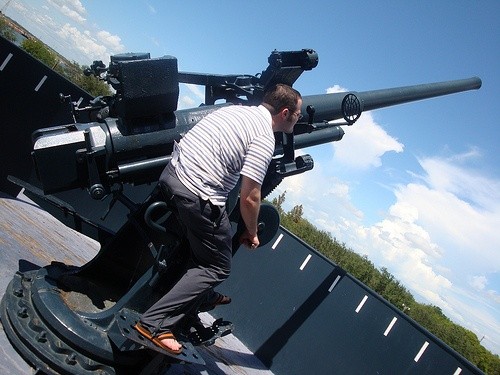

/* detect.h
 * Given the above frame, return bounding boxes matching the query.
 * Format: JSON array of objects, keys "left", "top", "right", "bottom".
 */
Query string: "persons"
[{"left": 134, "top": 84, "right": 303, "bottom": 354}]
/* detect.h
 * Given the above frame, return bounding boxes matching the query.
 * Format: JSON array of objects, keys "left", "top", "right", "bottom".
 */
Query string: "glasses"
[{"left": 288, "top": 109, "right": 303, "bottom": 120}]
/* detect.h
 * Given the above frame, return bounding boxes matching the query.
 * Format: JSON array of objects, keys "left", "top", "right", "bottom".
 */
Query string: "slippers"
[{"left": 134, "top": 321, "right": 183, "bottom": 354}]
